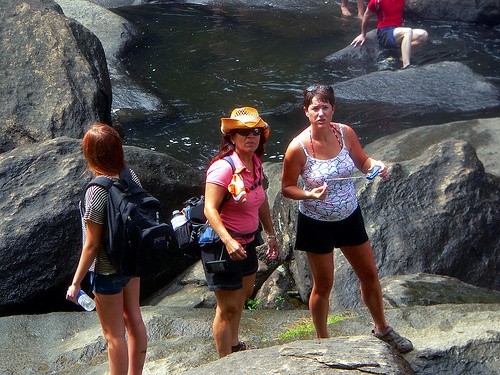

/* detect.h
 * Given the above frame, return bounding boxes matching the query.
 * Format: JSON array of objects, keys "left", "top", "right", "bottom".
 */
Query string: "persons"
[
  {"left": 341, "top": 1, "right": 364, "bottom": 20},
  {"left": 282, "top": 87, "right": 412, "bottom": 354},
  {"left": 66, "top": 124, "right": 148, "bottom": 375},
  {"left": 199, "top": 107, "right": 278, "bottom": 360},
  {"left": 351, "top": 0, "right": 428, "bottom": 70}
]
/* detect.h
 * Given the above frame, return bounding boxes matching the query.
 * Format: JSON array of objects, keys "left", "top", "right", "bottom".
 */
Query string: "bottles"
[
  {"left": 172, "top": 210, "right": 182, "bottom": 219},
  {"left": 68, "top": 285, "right": 96, "bottom": 312}
]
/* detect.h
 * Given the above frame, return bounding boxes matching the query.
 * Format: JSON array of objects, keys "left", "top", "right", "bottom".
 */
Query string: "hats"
[{"left": 221, "top": 107, "right": 270, "bottom": 140}]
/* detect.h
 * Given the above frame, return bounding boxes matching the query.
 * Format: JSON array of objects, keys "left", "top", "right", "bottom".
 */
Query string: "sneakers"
[{"left": 372, "top": 326, "right": 413, "bottom": 354}]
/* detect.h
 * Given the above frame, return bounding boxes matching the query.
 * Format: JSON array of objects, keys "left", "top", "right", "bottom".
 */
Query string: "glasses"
[{"left": 236, "top": 128, "right": 261, "bottom": 136}]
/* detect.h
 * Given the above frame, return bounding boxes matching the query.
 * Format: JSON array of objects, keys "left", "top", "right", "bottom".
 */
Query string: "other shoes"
[
  {"left": 232, "top": 342, "right": 257, "bottom": 352},
  {"left": 401, "top": 64, "right": 419, "bottom": 70}
]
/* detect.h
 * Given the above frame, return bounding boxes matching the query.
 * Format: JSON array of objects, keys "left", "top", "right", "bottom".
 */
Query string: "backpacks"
[
  {"left": 169, "top": 155, "right": 264, "bottom": 248},
  {"left": 82, "top": 166, "right": 170, "bottom": 274}
]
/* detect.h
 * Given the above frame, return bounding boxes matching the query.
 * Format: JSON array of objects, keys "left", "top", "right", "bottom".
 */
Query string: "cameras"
[{"left": 366, "top": 166, "right": 381, "bottom": 180}]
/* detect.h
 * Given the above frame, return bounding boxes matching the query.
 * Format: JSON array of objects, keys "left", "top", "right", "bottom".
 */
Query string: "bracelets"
[{"left": 266, "top": 235, "right": 276, "bottom": 238}]
